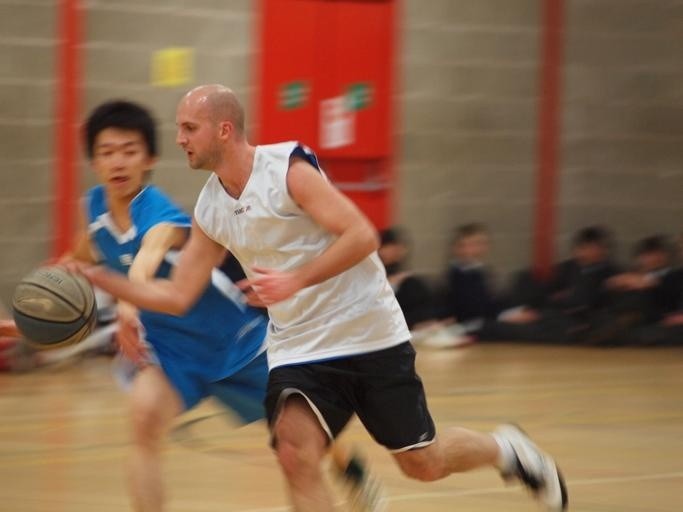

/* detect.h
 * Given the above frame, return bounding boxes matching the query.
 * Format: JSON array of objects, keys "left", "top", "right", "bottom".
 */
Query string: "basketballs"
[{"left": 13, "top": 265, "right": 97, "bottom": 350}]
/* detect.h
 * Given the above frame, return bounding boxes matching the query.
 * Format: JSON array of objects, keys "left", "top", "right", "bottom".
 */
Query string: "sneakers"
[{"left": 497, "top": 422, "right": 566, "bottom": 512}]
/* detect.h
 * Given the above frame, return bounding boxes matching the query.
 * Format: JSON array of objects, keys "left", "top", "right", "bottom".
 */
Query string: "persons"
[
  {"left": 46, "top": 84, "right": 567, "bottom": 512},
  {"left": 378, "top": 224, "right": 683, "bottom": 348},
  {"left": 0, "top": 101, "right": 381, "bottom": 512}
]
